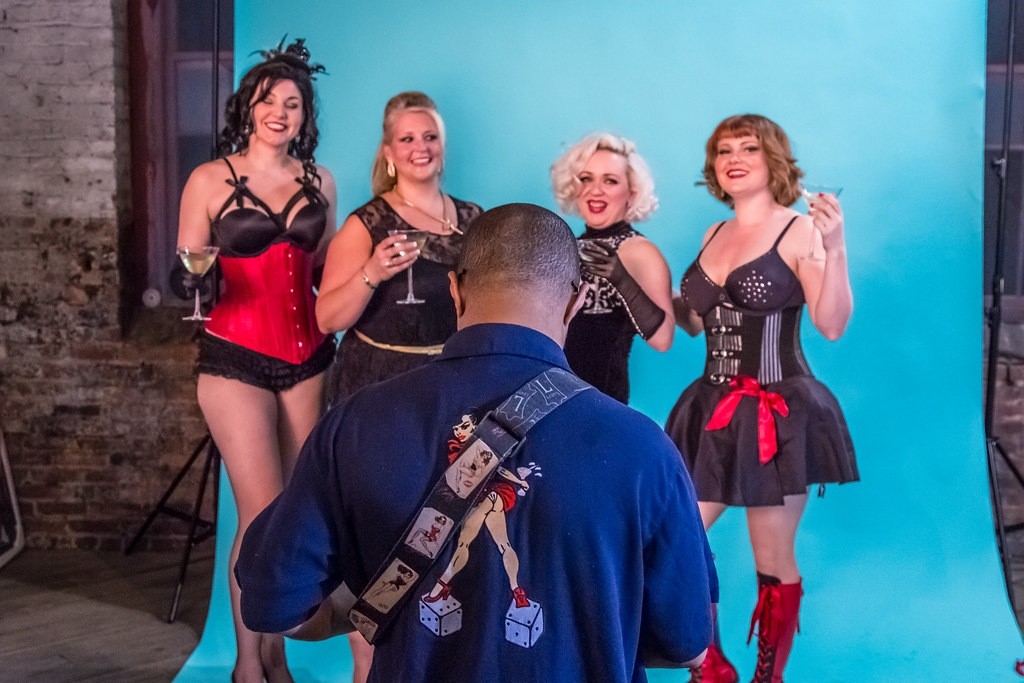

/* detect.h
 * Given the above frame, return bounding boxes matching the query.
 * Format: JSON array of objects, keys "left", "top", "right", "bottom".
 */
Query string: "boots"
[
  {"left": 747, "top": 571, "right": 803, "bottom": 683},
  {"left": 687, "top": 604, "right": 737, "bottom": 683}
]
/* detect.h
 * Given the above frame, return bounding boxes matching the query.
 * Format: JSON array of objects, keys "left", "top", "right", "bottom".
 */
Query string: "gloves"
[
  {"left": 169, "top": 254, "right": 209, "bottom": 300},
  {"left": 581, "top": 240, "right": 665, "bottom": 341}
]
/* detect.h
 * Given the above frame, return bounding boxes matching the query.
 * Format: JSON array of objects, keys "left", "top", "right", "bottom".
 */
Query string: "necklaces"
[{"left": 393, "top": 184, "right": 464, "bottom": 235}]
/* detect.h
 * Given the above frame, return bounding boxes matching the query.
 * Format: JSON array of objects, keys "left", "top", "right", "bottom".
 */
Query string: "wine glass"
[
  {"left": 796, "top": 183, "right": 843, "bottom": 262},
  {"left": 178, "top": 247, "right": 219, "bottom": 320},
  {"left": 577, "top": 240, "right": 615, "bottom": 314},
  {"left": 388, "top": 230, "right": 430, "bottom": 304}
]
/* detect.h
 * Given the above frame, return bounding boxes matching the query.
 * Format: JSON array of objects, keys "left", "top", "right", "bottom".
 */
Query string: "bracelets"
[{"left": 360, "top": 265, "right": 379, "bottom": 289}]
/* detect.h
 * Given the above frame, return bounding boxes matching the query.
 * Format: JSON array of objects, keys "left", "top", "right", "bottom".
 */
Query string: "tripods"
[
  {"left": 121, "top": 0, "right": 222, "bottom": 625},
  {"left": 985, "top": 0, "right": 1024, "bottom": 616}
]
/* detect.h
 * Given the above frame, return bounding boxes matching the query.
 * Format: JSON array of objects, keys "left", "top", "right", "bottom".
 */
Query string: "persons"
[
  {"left": 550, "top": 134, "right": 676, "bottom": 407},
  {"left": 170, "top": 36, "right": 336, "bottom": 683},
  {"left": 233, "top": 203, "right": 721, "bottom": 683},
  {"left": 665, "top": 114, "right": 860, "bottom": 683},
  {"left": 316, "top": 92, "right": 484, "bottom": 683}
]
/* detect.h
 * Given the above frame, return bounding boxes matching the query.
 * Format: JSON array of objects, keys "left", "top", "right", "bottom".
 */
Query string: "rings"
[{"left": 385, "top": 260, "right": 394, "bottom": 268}]
